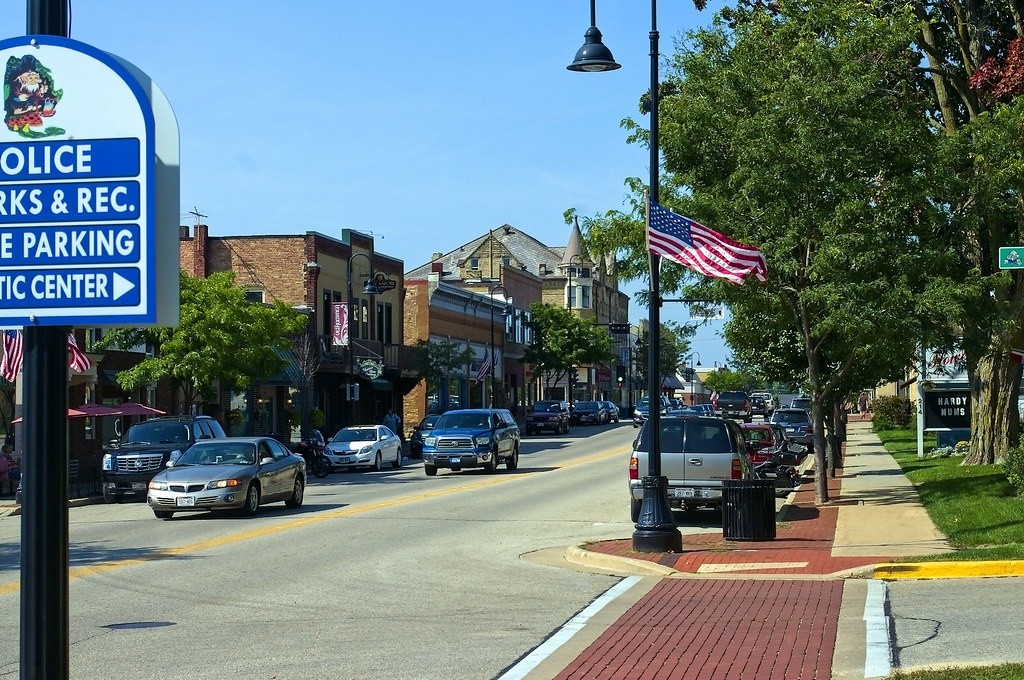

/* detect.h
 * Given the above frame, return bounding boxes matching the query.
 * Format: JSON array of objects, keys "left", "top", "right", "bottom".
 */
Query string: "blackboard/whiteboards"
[{"left": 923, "top": 389, "right": 972, "bottom": 431}]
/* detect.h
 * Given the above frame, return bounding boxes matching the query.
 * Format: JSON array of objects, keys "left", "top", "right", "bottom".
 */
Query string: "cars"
[
  {"left": 571, "top": 400, "right": 607, "bottom": 425},
  {"left": 667, "top": 396, "right": 714, "bottom": 415},
  {"left": 741, "top": 422, "right": 789, "bottom": 466},
  {"left": 600, "top": 401, "right": 620, "bottom": 422},
  {"left": 147, "top": 436, "right": 309, "bottom": 520},
  {"left": 750, "top": 396, "right": 770, "bottom": 418},
  {"left": 324, "top": 424, "right": 402, "bottom": 471}
]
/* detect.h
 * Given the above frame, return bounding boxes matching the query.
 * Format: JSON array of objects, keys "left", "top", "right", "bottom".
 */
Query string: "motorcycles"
[
  {"left": 301, "top": 438, "right": 332, "bottom": 478},
  {"left": 748, "top": 443, "right": 801, "bottom": 497}
]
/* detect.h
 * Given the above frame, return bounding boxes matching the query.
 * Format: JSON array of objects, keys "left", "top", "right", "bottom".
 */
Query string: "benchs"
[{"left": 0, "top": 457, "right": 80, "bottom": 498}]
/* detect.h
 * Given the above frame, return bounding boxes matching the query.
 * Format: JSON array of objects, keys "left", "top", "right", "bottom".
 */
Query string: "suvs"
[
  {"left": 422, "top": 408, "right": 520, "bottom": 475},
  {"left": 790, "top": 396, "right": 812, "bottom": 408},
  {"left": 410, "top": 413, "right": 443, "bottom": 459},
  {"left": 103, "top": 416, "right": 225, "bottom": 504},
  {"left": 714, "top": 391, "right": 751, "bottom": 421},
  {"left": 770, "top": 408, "right": 815, "bottom": 454},
  {"left": 628, "top": 416, "right": 754, "bottom": 522},
  {"left": 525, "top": 399, "right": 570, "bottom": 437}
]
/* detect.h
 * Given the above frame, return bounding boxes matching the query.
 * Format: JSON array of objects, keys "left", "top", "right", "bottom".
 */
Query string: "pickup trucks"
[
  {"left": 753, "top": 393, "right": 777, "bottom": 410},
  {"left": 633, "top": 395, "right": 670, "bottom": 428}
]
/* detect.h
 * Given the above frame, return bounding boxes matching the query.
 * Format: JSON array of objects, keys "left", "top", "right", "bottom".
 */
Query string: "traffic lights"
[
  {"left": 615, "top": 366, "right": 627, "bottom": 383},
  {"left": 570, "top": 368, "right": 579, "bottom": 383}
]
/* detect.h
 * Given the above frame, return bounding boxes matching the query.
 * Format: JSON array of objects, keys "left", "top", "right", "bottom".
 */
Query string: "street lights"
[
  {"left": 569, "top": 0, "right": 686, "bottom": 553},
  {"left": 490, "top": 286, "right": 513, "bottom": 408},
  {"left": 348, "top": 253, "right": 379, "bottom": 423},
  {"left": 568, "top": 253, "right": 585, "bottom": 401},
  {"left": 690, "top": 350, "right": 702, "bottom": 406},
  {"left": 627, "top": 323, "right": 644, "bottom": 417}
]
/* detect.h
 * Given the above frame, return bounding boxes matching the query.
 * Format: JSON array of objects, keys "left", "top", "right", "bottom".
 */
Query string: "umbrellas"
[{"left": 11, "top": 396, "right": 167, "bottom": 440}]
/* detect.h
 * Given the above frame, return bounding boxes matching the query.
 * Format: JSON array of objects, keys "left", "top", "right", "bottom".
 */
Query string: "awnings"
[
  {"left": 260, "top": 342, "right": 310, "bottom": 386},
  {"left": 644, "top": 376, "right": 685, "bottom": 390}
]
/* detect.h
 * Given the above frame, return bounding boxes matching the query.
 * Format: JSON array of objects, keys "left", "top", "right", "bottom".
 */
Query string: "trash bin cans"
[{"left": 721, "top": 478, "right": 779, "bottom": 542}]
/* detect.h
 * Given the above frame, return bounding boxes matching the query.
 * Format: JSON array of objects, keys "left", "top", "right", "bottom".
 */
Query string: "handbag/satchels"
[{"left": 858, "top": 404, "right": 862, "bottom": 411}]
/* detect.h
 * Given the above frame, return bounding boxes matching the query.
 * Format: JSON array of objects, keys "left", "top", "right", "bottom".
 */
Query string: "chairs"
[
  {"left": 711, "top": 432, "right": 731, "bottom": 452},
  {"left": 660, "top": 430, "right": 683, "bottom": 453}
]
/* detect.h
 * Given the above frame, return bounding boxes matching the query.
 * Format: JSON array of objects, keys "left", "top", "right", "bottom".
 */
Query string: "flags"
[
  {"left": 647, "top": 201, "right": 769, "bottom": 288},
  {"left": 710, "top": 388, "right": 720, "bottom": 410},
  {"left": 475, "top": 347, "right": 499, "bottom": 385},
  {"left": 0, "top": 329, "right": 23, "bottom": 382},
  {"left": 68, "top": 333, "right": 94, "bottom": 373},
  {"left": 332, "top": 302, "right": 348, "bottom": 346}
]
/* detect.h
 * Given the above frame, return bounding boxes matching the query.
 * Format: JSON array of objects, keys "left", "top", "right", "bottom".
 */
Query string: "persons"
[
  {"left": 858, "top": 393, "right": 868, "bottom": 419},
  {"left": 0, "top": 443, "right": 20, "bottom": 480},
  {"left": 383, "top": 407, "right": 400, "bottom": 435}
]
